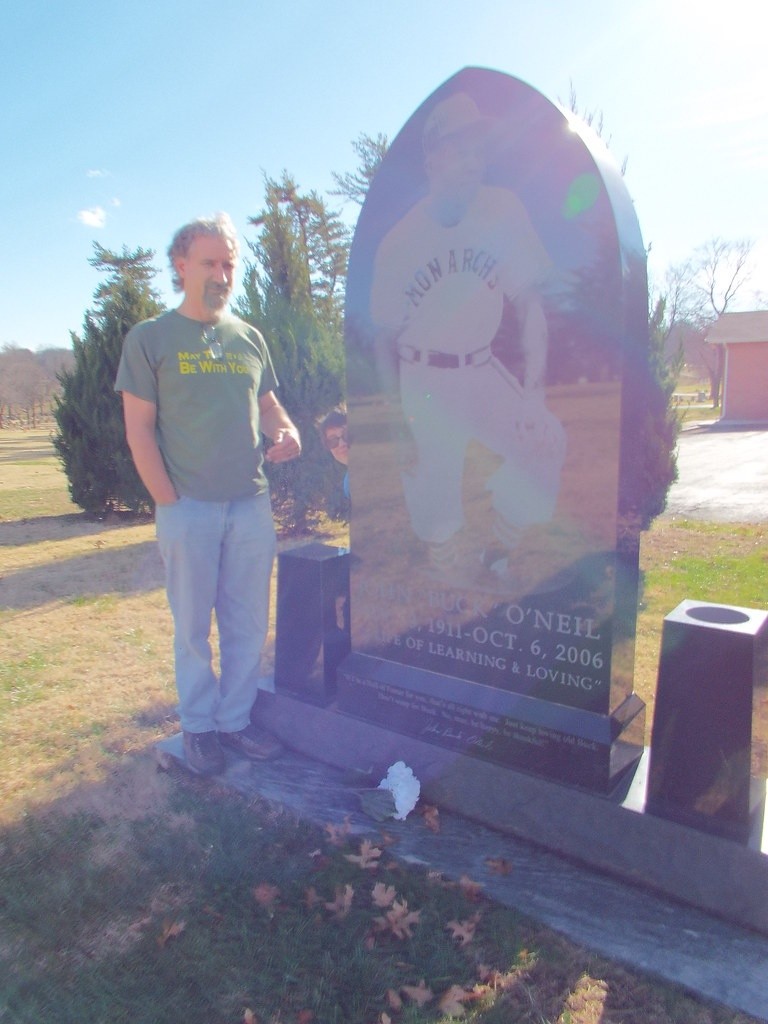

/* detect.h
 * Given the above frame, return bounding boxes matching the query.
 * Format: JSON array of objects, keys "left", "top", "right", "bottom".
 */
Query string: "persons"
[
  {"left": 320, "top": 412, "right": 350, "bottom": 500},
  {"left": 115, "top": 218, "right": 301, "bottom": 773}
]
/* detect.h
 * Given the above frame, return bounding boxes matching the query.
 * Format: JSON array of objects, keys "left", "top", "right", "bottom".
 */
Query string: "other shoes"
[
  {"left": 183, "top": 730, "right": 225, "bottom": 776},
  {"left": 217, "top": 725, "right": 283, "bottom": 761}
]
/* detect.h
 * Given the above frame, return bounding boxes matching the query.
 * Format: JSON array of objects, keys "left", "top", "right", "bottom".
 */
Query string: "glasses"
[{"left": 324, "top": 429, "right": 349, "bottom": 448}]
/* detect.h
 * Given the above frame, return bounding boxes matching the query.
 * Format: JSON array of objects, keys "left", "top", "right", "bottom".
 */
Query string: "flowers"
[{"left": 264, "top": 760, "right": 421, "bottom": 821}]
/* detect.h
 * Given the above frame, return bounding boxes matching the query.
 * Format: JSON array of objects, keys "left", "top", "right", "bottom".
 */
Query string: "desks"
[{"left": 673, "top": 393, "right": 697, "bottom": 405}]
